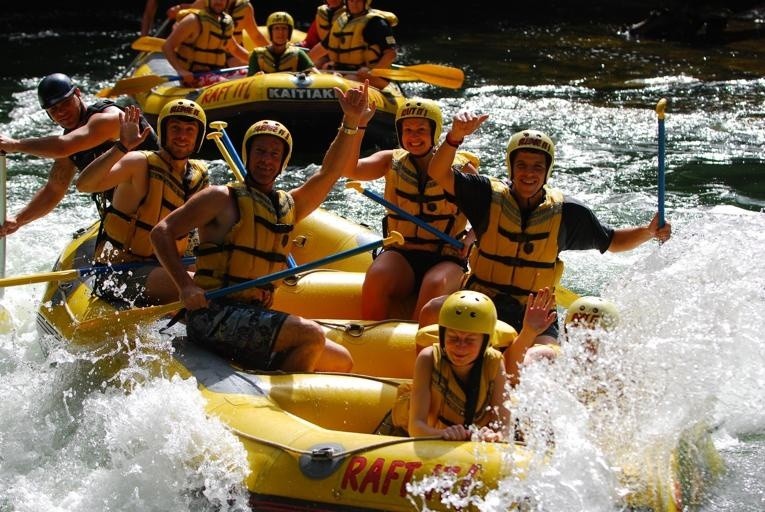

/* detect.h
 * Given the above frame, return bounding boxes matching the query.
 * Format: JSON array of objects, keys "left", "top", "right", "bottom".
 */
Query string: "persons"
[
  {"left": 289, "top": 0, "right": 347, "bottom": 69},
  {"left": 148, "top": 83, "right": 370, "bottom": 374},
  {"left": 159, "top": 0, "right": 250, "bottom": 89},
  {"left": 501, "top": 286, "right": 627, "bottom": 448},
  {"left": 305, "top": 0, "right": 399, "bottom": 90},
  {"left": 390, "top": 289, "right": 516, "bottom": 443},
  {"left": 165, "top": 0, "right": 270, "bottom": 68},
  {"left": 340, "top": 78, "right": 481, "bottom": 321},
  {"left": 139, "top": 0, "right": 195, "bottom": 38},
  {"left": 246, "top": 12, "right": 321, "bottom": 78},
  {"left": 74, "top": 99, "right": 209, "bottom": 321},
  {"left": 0, "top": 73, "right": 159, "bottom": 240},
  {"left": 414, "top": 109, "right": 672, "bottom": 357}
]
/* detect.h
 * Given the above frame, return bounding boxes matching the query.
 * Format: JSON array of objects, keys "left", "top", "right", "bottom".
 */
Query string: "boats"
[
  {"left": 37, "top": 204, "right": 719, "bottom": 512},
  {"left": 127, "top": 23, "right": 408, "bottom": 159}
]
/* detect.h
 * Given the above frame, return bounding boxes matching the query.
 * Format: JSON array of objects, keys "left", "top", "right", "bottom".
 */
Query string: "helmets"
[
  {"left": 37, "top": 73, "right": 77, "bottom": 109},
  {"left": 506, "top": 129, "right": 555, "bottom": 184},
  {"left": 438, "top": 290, "right": 498, "bottom": 359},
  {"left": 156, "top": 98, "right": 207, "bottom": 155},
  {"left": 241, "top": 120, "right": 293, "bottom": 174},
  {"left": 564, "top": 296, "right": 620, "bottom": 334},
  {"left": 266, "top": 12, "right": 294, "bottom": 42},
  {"left": 394, "top": 99, "right": 443, "bottom": 151}
]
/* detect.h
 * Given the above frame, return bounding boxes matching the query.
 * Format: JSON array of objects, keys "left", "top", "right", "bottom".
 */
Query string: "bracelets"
[
  {"left": 338, "top": 121, "right": 358, "bottom": 136},
  {"left": 445, "top": 131, "right": 464, "bottom": 149},
  {"left": 114, "top": 138, "right": 128, "bottom": 154},
  {"left": 358, "top": 126, "right": 368, "bottom": 130},
  {"left": 175, "top": 4, "right": 183, "bottom": 11}
]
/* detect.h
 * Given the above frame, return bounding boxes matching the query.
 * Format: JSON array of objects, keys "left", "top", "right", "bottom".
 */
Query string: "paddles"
[
  {"left": 132, "top": 36, "right": 178, "bottom": 52},
  {"left": 112, "top": 65, "right": 250, "bottom": 94},
  {"left": 76, "top": 230, "right": 404, "bottom": 346},
  {"left": 345, "top": 182, "right": 578, "bottom": 309},
  {"left": 95, "top": 18, "right": 171, "bottom": 98},
  {"left": 390, "top": 64, "right": 464, "bottom": 89},
  {"left": 0, "top": 256, "right": 194, "bottom": 286},
  {"left": 319, "top": 68, "right": 418, "bottom": 81}
]
